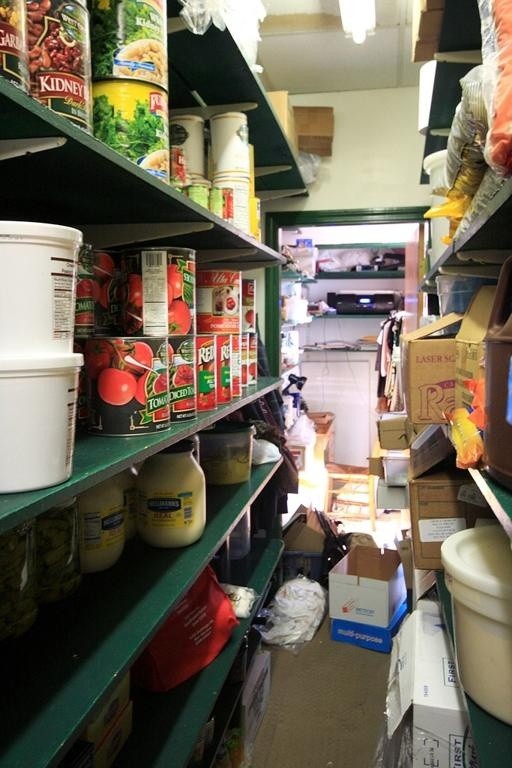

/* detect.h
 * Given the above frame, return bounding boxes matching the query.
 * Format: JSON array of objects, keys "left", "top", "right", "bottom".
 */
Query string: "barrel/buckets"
[
  {"left": 200, "top": 420, "right": 255, "bottom": 485},
  {"left": 482, "top": 335, "right": 512, "bottom": 488},
  {"left": 421, "top": 147, "right": 450, "bottom": 268},
  {"left": 442, "top": 526, "right": 512, "bottom": 726},
  {"left": 0, "top": 219, "right": 84, "bottom": 360},
  {"left": 0, "top": 354, "right": 86, "bottom": 494}
]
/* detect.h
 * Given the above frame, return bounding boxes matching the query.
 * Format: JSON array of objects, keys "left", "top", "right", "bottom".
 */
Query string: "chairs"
[{"left": 327, "top": 471, "right": 386, "bottom": 531}]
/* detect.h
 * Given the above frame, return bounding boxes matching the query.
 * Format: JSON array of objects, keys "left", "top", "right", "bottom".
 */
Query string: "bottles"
[
  {"left": 170, "top": 112, "right": 261, "bottom": 241},
  {"left": 74, "top": 440, "right": 206, "bottom": 576}
]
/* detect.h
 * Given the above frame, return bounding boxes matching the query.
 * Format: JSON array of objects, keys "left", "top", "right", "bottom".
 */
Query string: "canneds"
[
  {"left": 72, "top": 245, "right": 259, "bottom": 437},
  {"left": 0, "top": 1, "right": 171, "bottom": 185},
  {"left": 171, "top": 112, "right": 264, "bottom": 242}
]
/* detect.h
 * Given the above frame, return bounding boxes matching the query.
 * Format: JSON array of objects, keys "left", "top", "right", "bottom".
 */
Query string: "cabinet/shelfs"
[
  {"left": 410, "top": 1, "right": 512, "bottom": 766},
  {"left": 0, "top": 2, "right": 286, "bottom": 768},
  {"left": 302, "top": 269, "right": 404, "bottom": 318}
]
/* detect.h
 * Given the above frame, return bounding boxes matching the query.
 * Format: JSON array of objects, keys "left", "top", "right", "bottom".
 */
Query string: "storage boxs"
[
  {"left": 378, "top": 286, "right": 499, "bottom": 577},
  {"left": 281, "top": 506, "right": 412, "bottom": 654}
]
